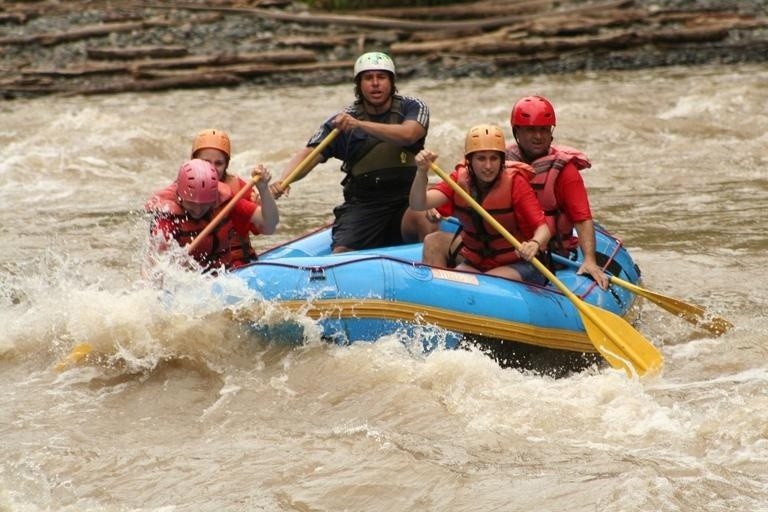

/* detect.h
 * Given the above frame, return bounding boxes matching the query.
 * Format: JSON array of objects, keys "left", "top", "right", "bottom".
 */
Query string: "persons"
[
  {"left": 267, "top": 52, "right": 440, "bottom": 255},
  {"left": 140, "top": 159, "right": 279, "bottom": 282},
  {"left": 172, "top": 129, "right": 261, "bottom": 268},
  {"left": 422, "top": 96, "right": 609, "bottom": 292},
  {"left": 409, "top": 124, "right": 555, "bottom": 290}
]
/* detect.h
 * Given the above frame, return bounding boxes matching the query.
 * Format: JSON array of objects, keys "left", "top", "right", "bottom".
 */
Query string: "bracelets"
[{"left": 530, "top": 238, "right": 541, "bottom": 247}]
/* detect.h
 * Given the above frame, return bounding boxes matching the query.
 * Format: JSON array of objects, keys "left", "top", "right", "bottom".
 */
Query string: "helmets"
[
  {"left": 511, "top": 95, "right": 556, "bottom": 129},
  {"left": 464, "top": 124, "right": 507, "bottom": 156},
  {"left": 191, "top": 128, "right": 231, "bottom": 159},
  {"left": 353, "top": 51, "right": 395, "bottom": 80},
  {"left": 177, "top": 159, "right": 218, "bottom": 205}
]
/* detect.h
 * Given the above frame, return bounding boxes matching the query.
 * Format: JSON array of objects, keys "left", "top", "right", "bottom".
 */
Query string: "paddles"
[
  {"left": 546, "top": 250, "right": 734, "bottom": 340},
  {"left": 430, "top": 163, "right": 664, "bottom": 380},
  {"left": 68, "top": 176, "right": 260, "bottom": 361}
]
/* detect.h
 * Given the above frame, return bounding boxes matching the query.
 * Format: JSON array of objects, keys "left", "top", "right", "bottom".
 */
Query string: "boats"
[{"left": 208, "top": 222, "right": 641, "bottom": 369}]
